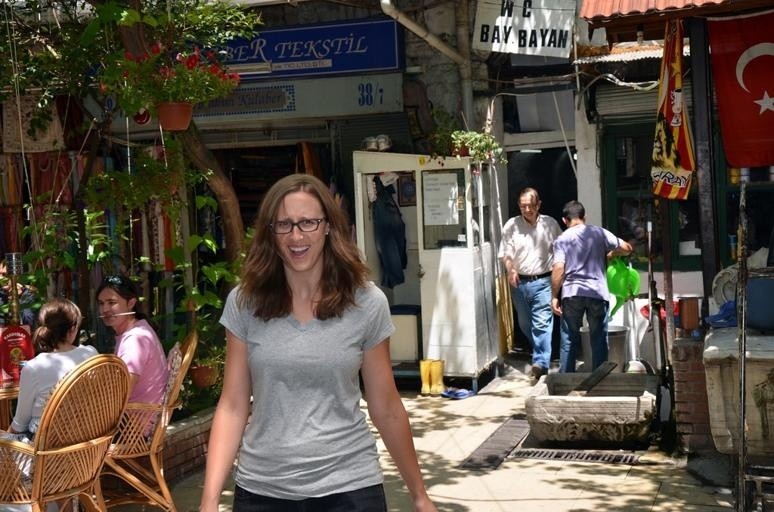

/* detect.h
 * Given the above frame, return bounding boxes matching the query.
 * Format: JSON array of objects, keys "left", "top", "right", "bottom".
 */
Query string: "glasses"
[{"left": 266, "top": 215, "right": 326, "bottom": 233}]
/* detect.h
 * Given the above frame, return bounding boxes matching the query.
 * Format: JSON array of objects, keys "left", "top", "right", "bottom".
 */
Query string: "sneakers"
[{"left": 532, "top": 363, "right": 548, "bottom": 379}]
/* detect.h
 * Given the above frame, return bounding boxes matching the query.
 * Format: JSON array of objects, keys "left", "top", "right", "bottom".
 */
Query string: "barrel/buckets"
[{"left": 580, "top": 326, "right": 630, "bottom": 373}]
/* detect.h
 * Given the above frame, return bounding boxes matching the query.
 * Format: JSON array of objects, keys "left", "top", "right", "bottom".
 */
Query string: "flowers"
[{"left": 124, "top": 40, "right": 239, "bottom": 108}]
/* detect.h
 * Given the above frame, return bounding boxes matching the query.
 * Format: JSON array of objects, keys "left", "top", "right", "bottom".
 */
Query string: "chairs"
[
  {"left": 0, "top": 353, "right": 132, "bottom": 512},
  {"left": 78, "top": 329, "right": 199, "bottom": 512}
]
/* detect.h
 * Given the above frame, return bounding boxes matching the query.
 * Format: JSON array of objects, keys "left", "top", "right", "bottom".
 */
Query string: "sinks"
[{"left": 526, "top": 373, "right": 655, "bottom": 424}]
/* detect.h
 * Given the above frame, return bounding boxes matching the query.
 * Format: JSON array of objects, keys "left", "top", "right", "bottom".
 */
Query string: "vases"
[{"left": 155, "top": 103, "right": 193, "bottom": 131}]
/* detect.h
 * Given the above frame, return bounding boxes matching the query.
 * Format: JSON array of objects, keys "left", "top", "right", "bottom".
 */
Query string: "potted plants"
[
  {"left": 451, "top": 129, "right": 509, "bottom": 176},
  {"left": 190, "top": 354, "right": 223, "bottom": 387},
  {"left": 422, "top": 103, "right": 461, "bottom": 160}
]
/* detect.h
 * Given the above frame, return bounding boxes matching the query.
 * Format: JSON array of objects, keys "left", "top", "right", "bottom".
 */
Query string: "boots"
[
  {"left": 418, "top": 358, "right": 431, "bottom": 396},
  {"left": 431, "top": 359, "right": 449, "bottom": 396}
]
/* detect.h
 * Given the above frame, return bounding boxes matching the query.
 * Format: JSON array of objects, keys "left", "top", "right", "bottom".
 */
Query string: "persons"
[
  {"left": 95, "top": 278, "right": 168, "bottom": 443},
  {"left": 550, "top": 199, "right": 633, "bottom": 373},
  {"left": 196, "top": 174, "right": 436, "bottom": 512},
  {"left": 497, "top": 187, "right": 564, "bottom": 380},
  {"left": 0, "top": 296, "right": 100, "bottom": 512}
]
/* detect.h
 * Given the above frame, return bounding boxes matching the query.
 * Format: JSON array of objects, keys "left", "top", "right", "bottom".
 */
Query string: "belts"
[{"left": 515, "top": 271, "right": 552, "bottom": 281}]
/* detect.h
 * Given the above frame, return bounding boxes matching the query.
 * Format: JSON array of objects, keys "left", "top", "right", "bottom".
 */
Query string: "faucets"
[{"left": 636, "top": 358, "right": 657, "bottom": 375}]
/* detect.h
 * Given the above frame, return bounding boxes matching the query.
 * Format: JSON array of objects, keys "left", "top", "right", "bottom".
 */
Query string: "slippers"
[
  {"left": 441, "top": 385, "right": 457, "bottom": 397},
  {"left": 706, "top": 300, "right": 736, "bottom": 321},
  {"left": 452, "top": 388, "right": 473, "bottom": 399},
  {"left": 710, "top": 319, "right": 738, "bottom": 328}
]
